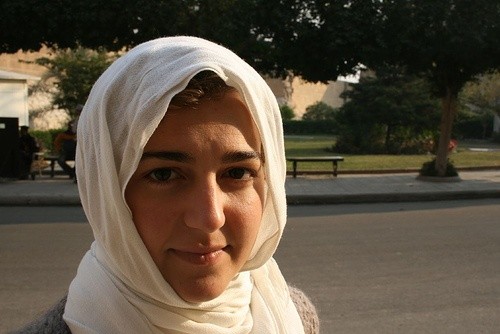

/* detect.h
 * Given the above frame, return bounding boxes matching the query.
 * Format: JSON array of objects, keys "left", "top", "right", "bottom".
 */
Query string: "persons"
[
  {"left": 54, "top": 121, "right": 77, "bottom": 183},
  {"left": 14, "top": 126, "right": 40, "bottom": 180},
  {"left": 14, "top": 36, "right": 319, "bottom": 334}
]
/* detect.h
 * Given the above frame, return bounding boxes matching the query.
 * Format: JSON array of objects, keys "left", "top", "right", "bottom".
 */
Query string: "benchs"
[
  {"left": 44, "top": 156, "right": 75, "bottom": 177},
  {"left": 286, "top": 156, "right": 344, "bottom": 178}
]
